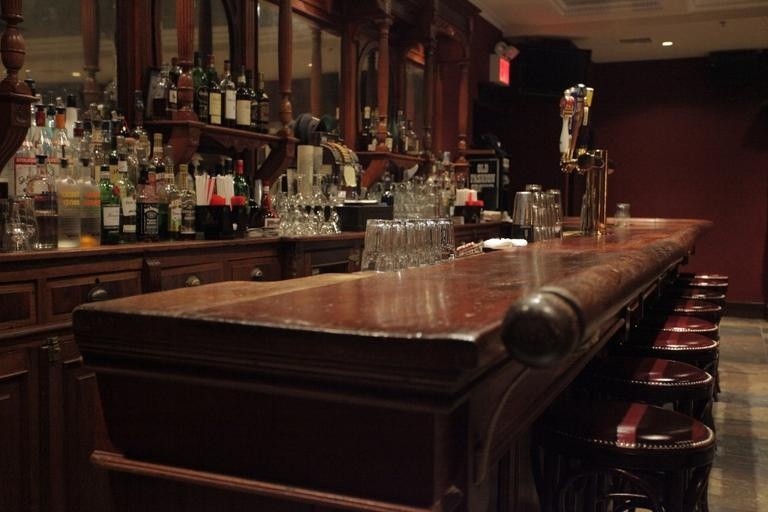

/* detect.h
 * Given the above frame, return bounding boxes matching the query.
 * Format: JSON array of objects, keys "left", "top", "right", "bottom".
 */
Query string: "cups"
[
  {"left": 508, "top": 182, "right": 563, "bottom": 242},
  {"left": 361, "top": 174, "right": 456, "bottom": 272},
  {"left": 613, "top": 202, "right": 632, "bottom": 229}
]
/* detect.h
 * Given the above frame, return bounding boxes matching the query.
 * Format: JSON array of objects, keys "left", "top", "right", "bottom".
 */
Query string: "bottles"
[
  {"left": 2, "top": 50, "right": 270, "bottom": 253},
  {"left": 359, "top": 102, "right": 420, "bottom": 155}
]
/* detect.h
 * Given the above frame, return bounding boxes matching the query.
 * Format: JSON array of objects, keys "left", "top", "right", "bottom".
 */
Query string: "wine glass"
[{"left": 271, "top": 174, "right": 343, "bottom": 237}]
